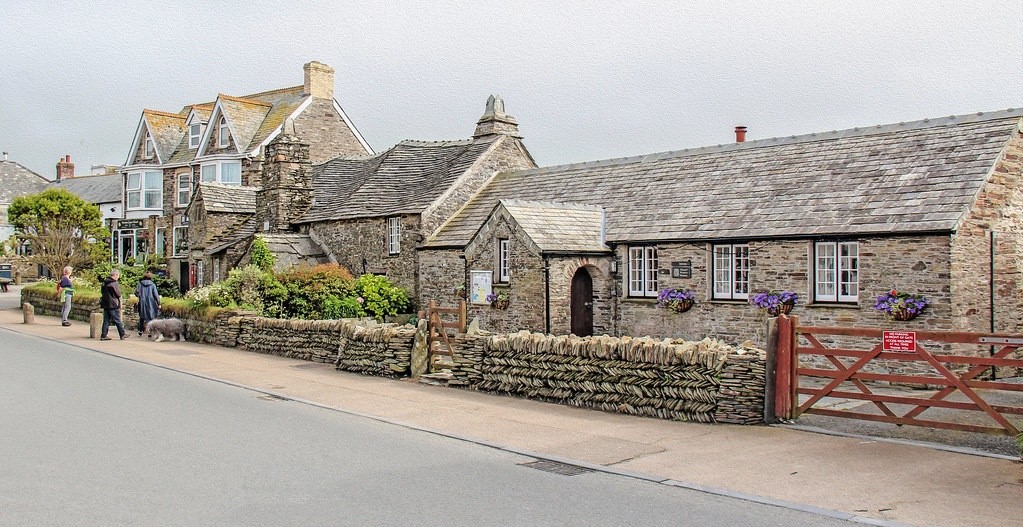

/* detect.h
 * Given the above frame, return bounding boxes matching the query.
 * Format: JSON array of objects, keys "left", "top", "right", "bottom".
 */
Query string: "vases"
[
  {"left": 492, "top": 301, "right": 509, "bottom": 312},
  {"left": 757, "top": 302, "right": 794, "bottom": 317},
  {"left": 662, "top": 300, "right": 695, "bottom": 314},
  {"left": 887, "top": 308, "right": 919, "bottom": 321}
]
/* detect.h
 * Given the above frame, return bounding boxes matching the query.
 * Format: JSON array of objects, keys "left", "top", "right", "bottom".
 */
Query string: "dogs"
[{"left": 145, "top": 317, "right": 186, "bottom": 342}]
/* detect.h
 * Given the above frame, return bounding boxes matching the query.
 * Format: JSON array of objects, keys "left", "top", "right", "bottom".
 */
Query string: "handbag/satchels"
[{"left": 134, "top": 303, "right": 140, "bottom": 313}]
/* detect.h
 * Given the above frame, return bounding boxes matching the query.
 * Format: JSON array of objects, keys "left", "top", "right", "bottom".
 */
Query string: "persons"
[
  {"left": 100, "top": 270, "right": 131, "bottom": 341},
  {"left": 138, "top": 271, "right": 162, "bottom": 336},
  {"left": 60, "top": 266, "right": 74, "bottom": 326}
]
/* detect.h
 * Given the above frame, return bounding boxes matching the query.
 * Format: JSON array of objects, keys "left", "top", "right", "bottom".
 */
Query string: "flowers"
[
  {"left": 486, "top": 292, "right": 511, "bottom": 310},
  {"left": 873, "top": 291, "right": 930, "bottom": 324},
  {"left": 657, "top": 289, "right": 700, "bottom": 316},
  {"left": 747, "top": 290, "right": 798, "bottom": 323}
]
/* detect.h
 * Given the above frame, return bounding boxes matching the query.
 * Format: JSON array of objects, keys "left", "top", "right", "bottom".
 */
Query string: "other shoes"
[
  {"left": 147, "top": 334, "right": 152, "bottom": 339},
  {"left": 137, "top": 331, "right": 143, "bottom": 336},
  {"left": 100, "top": 336, "right": 112, "bottom": 340},
  {"left": 62, "top": 322, "right": 71, "bottom": 326},
  {"left": 120, "top": 333, "right": 130, "bottom": 340}
]
